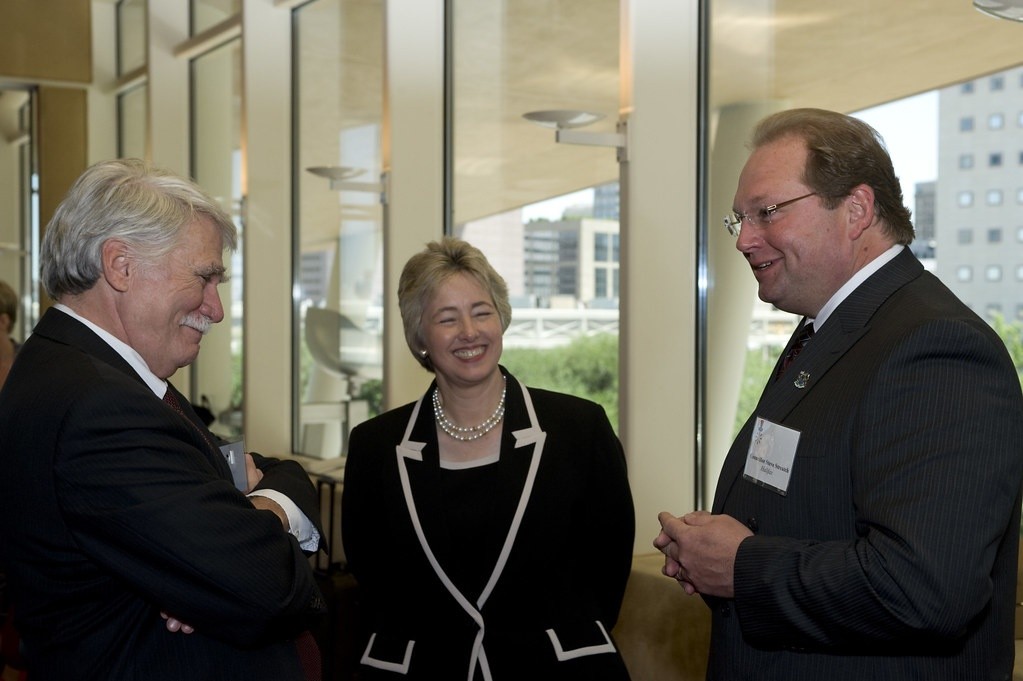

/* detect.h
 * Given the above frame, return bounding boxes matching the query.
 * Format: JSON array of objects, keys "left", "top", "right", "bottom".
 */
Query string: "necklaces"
[{"left": 433, "top": 375, "right": 506, "bottom": 440}]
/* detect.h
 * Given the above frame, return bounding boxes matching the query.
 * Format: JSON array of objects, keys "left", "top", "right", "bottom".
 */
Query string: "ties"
[
  {"left": 773, "top": 322, "right": 816, "bottom": 382},
  {"left": 163, "top": 387, "right": 184, "bottom": 418}
]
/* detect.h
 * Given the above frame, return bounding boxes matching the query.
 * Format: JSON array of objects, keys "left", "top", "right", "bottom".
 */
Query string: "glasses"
[{"left": 723, "top": 192, "right": 817, "bottom": 237}]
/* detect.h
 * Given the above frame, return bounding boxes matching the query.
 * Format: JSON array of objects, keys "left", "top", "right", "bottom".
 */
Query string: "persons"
[
  {"left": 341, "top": 237, "right": 636, "bottom": 681},
  {"left": 652, "top": 108, "right": 1023, "bottom": 681},
  {"left": 0, "top": 159, "right": 322, "bottom": 681},
  {"left": 0, "top": 280, "right": 22, "bottom": 393}
]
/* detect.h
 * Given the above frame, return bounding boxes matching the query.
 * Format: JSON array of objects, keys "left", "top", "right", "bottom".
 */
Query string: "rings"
[{"left": 675, "top": 567, "right": 682, "bottom": 582}]
